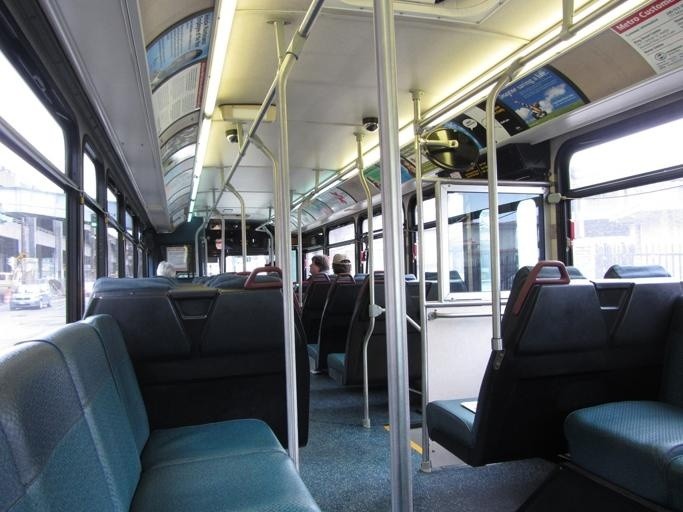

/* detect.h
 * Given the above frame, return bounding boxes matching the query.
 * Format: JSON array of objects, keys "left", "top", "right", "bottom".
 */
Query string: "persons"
[
  {"left": 328, "top": 253, "right": 354, "bottom": 282},
  {"left": 305, "top": 253, "right": 329, "bottom": 280},
  {"left": 156, "top": 260, "right": 176, "bottom": 278}
]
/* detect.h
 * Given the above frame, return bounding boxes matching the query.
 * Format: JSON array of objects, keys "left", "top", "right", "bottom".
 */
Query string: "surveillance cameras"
[
  {"left": 225, "top": 129, "right": 238, "bottom": 144},
  {"left": 363, "top": 117, "right": 379, "bottom": 132}
]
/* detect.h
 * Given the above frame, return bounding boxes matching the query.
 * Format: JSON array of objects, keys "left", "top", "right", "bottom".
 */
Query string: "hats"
[{"left": 333, "top": 253, "right": 352, "bottom": 265}]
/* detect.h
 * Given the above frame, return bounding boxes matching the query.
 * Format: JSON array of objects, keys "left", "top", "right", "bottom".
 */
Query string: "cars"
[{"left": 9, "top": 285, "right": 50, "bottom": 310}]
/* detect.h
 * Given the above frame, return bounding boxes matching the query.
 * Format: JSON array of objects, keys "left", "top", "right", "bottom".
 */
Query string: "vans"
[{"left": 0, "top": 272, "right": 21, "bottom": 304}]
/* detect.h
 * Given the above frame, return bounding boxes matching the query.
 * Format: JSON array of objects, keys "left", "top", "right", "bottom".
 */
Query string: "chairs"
[
  {"left": 425, "top": 258, "right": 683, "bottom": 511},
  {"left": 1, "top": 312, "right": 320, "bottom": 512},
  {"left": 298, "top": 269, "right": 473, "bottom": 431},
  {"left": 80, "top": 268, "right": 310, "bottom": 454}
]
